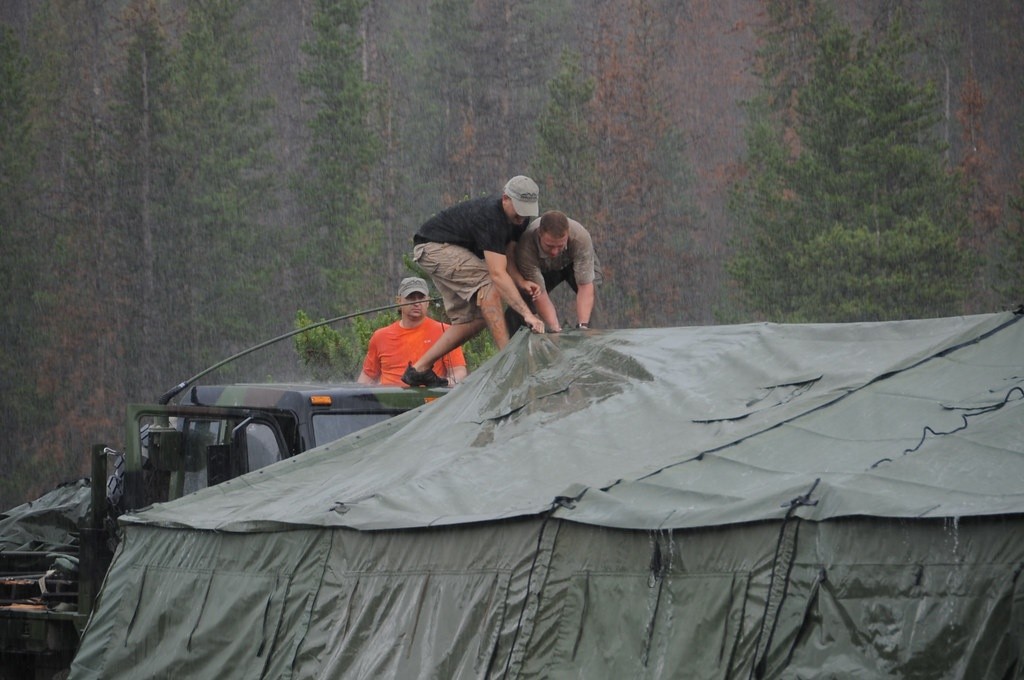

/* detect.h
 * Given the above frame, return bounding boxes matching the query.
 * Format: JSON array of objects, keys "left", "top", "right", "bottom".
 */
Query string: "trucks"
[{"left": 0, "top": 371, "right": 467, "bottom": 680}]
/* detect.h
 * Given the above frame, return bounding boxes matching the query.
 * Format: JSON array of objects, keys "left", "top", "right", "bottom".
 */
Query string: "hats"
[
  {"left": 502, "top": 174, "right": 541, "bottom": 217},
  {"left": 397, "top": 277, "right": 430, "bottom": 298}
]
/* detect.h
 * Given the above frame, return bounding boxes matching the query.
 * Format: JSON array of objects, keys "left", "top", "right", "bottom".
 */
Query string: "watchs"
[{"left": 576, "top": 321, "right": 592, "bottom": 329}]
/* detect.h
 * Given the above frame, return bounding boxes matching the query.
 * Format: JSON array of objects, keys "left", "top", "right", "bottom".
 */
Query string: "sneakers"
[{"left": 401, "top": 361, "right": 449, "bottom": 388}]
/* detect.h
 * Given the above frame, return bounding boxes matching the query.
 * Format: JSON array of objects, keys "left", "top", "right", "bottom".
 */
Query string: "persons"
[
  {"left": 498, "top": 209, "right": 607, "bottom": 355},
  {"left": 400, "top": 174, "right": 546, "bottom": 389},
  {"left": 354, "top": 276, "right": 467, "bottom": 388}
]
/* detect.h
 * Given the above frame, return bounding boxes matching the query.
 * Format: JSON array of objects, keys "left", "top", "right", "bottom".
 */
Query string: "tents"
[{"left": 65, "top": 304, "right": 1024, "bottom": 680}]
